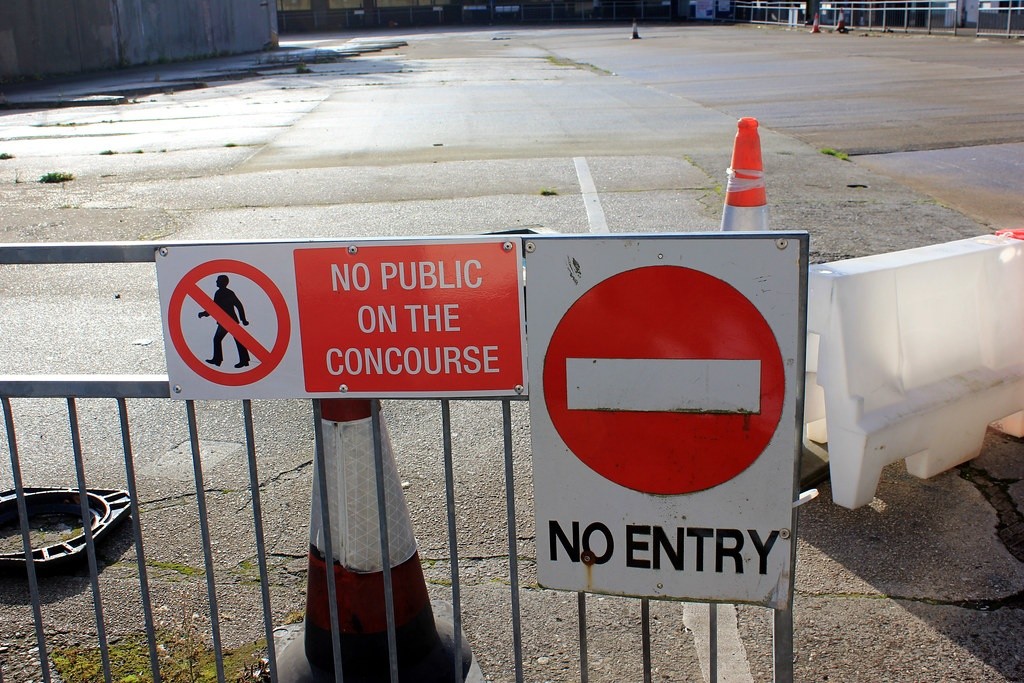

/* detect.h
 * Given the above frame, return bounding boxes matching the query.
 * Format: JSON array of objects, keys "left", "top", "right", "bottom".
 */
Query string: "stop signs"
[{"left": 522, "top": 229, "right": 808, "bottom": 611}]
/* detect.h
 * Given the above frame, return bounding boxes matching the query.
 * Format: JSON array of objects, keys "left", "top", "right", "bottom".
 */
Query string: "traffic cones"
[
  {"left": 718, "top": 116, "right": 772, "bottom": 232},
  {"left": 834, "top": 8, "right": 846, "bottom": 31},
  {"left": 630, "top": 17, "right": 642, "bottom": 39},
  {"left": 275, "top": 400, "right": 473, "bottom": 683},
  {"left": 810, "top": 13, "right": 821, "bottom": 33}
]
